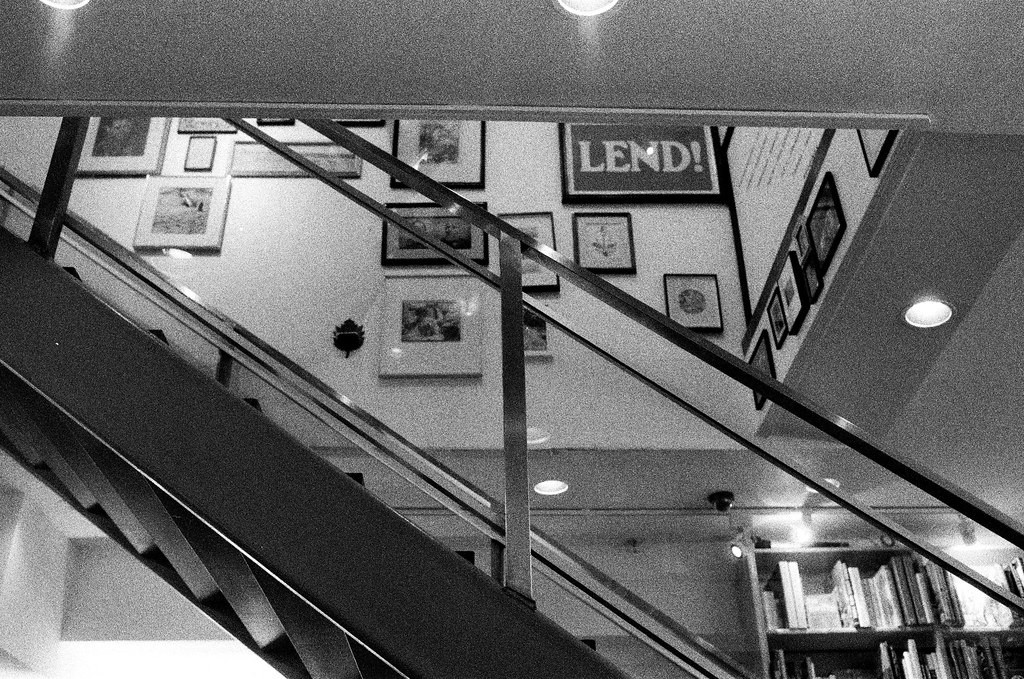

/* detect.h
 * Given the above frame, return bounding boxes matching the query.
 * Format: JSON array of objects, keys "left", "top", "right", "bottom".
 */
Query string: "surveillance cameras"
[{"left": 708, "top": 491, "right": 735, "bottom": 512}]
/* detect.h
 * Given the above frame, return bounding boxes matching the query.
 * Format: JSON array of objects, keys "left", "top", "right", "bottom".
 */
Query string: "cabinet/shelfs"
[{"left": 735, "top": 544, "right": 1024, "bottom": 679}]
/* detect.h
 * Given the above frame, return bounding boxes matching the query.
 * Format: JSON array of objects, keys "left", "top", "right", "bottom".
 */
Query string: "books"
[{"left": 761, "top": 555, "right": 1024, "bottom": 679}]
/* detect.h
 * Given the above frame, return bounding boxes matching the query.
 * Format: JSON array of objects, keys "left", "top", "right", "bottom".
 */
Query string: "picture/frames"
[
  {"left": 184, "top": 136, "right": 217, "bottom": 171},
  {"left": 571, "top": 212, "right": 637, "bottom": 276},
  {"left": 132, "top": 174, "right": 233, "bottom": 252},
  {"left": 558, "top": 122, "right": 731, "bottom": 206},
  {"left": 663, "top": 274, "right": 724, "bottom": 333},
  {"left": 497, "top": 211, "right": 561, "bottom": 294},
  {"left": 379, "top": 278, "right": 484, "bottom": 377},
  {"left": 522, "top": 302, "right": 555, "bottom": 357},
  {"left": 257, "top": 118, "right": 296, "bottom": 126},
  {"left": 177, "top": 117, "right": 237, "bottom": 134},
  {"left": 332, "top": 119, "right": 386, "bottom": 127},
  {"left": 748, "top": 329, "right": 777, "bottom": 410},
  {"left": 74, "top": 116, "right": 172, "bottom": 178},
  {"left": 802, "top": 249, "right": 825, "bottom": 304},
  {"left": 776, "top": 251, "right": 810, "bottom": 336},
  {"left": 804, "top": 171, "right": 848, "bottom": 275},
  {"left": 390, "top": 119, "right": 486, "bottom": 189},
  {"left": 380, "top": 202, "right": 489, "bottom": 267},
  {"left": 855, "top": 128, "right": 899, "bottom": 178},
  {"left": 766, "top": 286, "right": 789, "bottom": 350},
  {"left": 225, "top": 140, "right": 363, "bottom": 178}
]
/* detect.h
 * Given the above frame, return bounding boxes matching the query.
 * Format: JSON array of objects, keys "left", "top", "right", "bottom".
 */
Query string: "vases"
[{"left": 791, "top": 216, "right": 812, "bottom": 266}]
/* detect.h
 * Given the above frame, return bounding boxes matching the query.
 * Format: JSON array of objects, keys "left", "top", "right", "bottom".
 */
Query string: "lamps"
[
  {"left": 727, "top": 527, "right": 754, "bottom": 562},
  {"left": 958, "top": 515, "right": 978, "bottom": 546}
]
